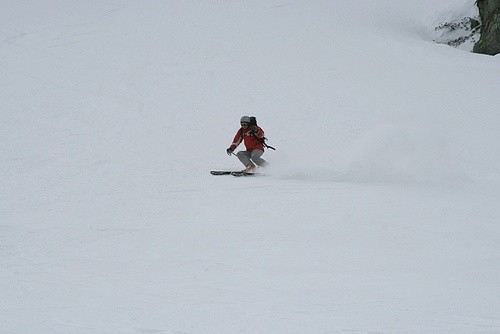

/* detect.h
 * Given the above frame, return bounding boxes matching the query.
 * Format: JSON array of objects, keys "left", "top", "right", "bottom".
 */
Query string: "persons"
[{"left": 227, "top": 116, "right": 269, "bottom": 172}]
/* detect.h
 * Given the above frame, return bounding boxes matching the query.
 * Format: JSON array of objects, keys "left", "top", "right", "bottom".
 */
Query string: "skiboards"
[{"left": 210, "top": 168, "right": 259, "bottom": 176}]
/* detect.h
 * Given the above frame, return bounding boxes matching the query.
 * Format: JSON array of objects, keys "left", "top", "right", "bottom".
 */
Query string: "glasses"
[{"left": 241, "top": 122, "right": 249, "bottom": 126}]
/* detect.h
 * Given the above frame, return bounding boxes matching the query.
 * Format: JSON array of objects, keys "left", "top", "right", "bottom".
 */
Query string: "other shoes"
[{"left": 243, "top": 163, "right": 256, "bottom": 172}]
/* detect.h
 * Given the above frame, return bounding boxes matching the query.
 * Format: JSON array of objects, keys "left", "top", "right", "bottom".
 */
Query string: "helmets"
[{"left": 240, "top": 116, "right": 250, "bottom": 123}]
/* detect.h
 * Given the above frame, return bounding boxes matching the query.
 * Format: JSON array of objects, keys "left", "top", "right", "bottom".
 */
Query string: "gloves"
[
  {"left": 227, "top": 147, "right": 234, "bottom": 156},
  {"left": 250, "top": 127, "right": 258, "bottom": 135}
]
